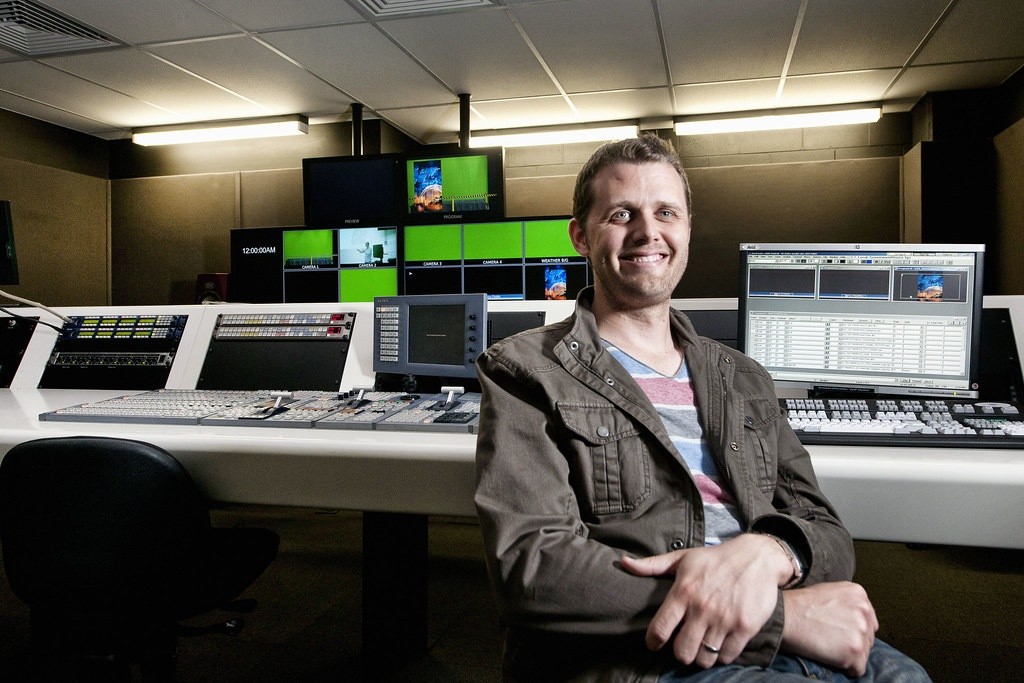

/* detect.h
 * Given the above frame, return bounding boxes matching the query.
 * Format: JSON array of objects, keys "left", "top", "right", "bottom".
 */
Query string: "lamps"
[
  {"left": 674, "top": 100, "right": 882, "bottom": 136},
  {"left": 131, "top": 113, "right": 309, "bottom": 147},
  {"left": 459, "top": 117, "right": 640, "bottom": 148}
]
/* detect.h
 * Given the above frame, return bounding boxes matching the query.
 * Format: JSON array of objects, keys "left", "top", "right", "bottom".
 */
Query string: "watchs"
[{"left": 762, "top": 533, "right": 808, "bottom": 589}]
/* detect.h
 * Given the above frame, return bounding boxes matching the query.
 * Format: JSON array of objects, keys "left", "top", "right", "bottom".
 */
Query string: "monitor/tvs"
[
  {"left": 230, "top": 146, "right": 597, "bottom": 304},
  {"left": 409, "top": 304, "right": 467, "bottom": 365},
  {"left": 738, "top": 242, "right": 986, "bottom": 400}
]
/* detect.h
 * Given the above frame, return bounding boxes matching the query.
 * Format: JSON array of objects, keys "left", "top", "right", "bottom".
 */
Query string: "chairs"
[{"left": 0, "top": 436, "right": 281, "bottom": 683}]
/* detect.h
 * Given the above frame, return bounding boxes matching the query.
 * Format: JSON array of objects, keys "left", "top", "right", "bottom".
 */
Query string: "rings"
[{"left": 701, "top": 640, "right": 721, "bottom": 653}]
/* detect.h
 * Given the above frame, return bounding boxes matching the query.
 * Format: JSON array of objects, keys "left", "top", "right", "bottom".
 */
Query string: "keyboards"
[{"left": 780, "top": 399, "right": 1024, "bottom": 446}]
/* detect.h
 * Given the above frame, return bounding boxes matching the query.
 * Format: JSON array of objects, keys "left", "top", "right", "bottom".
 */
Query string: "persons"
[
  {"left": 357, "top": 242, "right": 372, "bottom": 263},
  {"left": 473, "top": 136, "right": 931, "bottom": 683}
]
